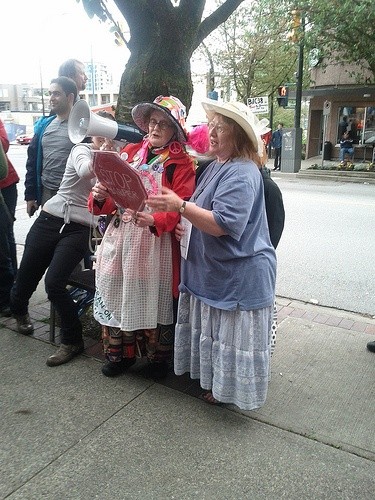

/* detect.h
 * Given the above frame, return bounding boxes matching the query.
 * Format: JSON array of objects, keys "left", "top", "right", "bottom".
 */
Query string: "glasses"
[
  {"left": 149, "top": 118, "right": 171, "bottom": 130},
  {"left": 208, "top": 121, "right": 234, "bottom": 134}
]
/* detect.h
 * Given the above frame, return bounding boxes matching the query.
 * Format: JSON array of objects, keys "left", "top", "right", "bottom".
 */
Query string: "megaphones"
[{"left": 66, "top": 99, "right": 144, "bottom": 144}]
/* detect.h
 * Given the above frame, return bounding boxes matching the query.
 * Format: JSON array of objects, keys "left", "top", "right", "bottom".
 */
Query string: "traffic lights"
[{"left": 277, "top": 86, "right": 288, "bottom": 106}]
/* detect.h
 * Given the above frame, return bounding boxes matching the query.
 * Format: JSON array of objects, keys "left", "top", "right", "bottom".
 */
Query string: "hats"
[
  {"left": 130, "top": 95, "right": 188, "bottom": 144},
  {"left": 200, "top": 99, "right": 271, "bottom": 159}
]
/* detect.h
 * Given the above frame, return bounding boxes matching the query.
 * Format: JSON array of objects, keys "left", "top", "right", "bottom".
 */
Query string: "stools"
[{"left": 49, "top": 270, "right": 95, "bottom": 342}]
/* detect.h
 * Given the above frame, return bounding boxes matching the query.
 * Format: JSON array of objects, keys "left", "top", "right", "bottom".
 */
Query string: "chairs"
[{"left": 340, "top": 145, "right": 355, "bottom": 162}]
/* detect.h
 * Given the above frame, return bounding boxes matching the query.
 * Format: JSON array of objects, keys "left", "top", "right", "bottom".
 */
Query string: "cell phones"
[{"left": 30, "top": 206, "right": 36, "bottom": 215}]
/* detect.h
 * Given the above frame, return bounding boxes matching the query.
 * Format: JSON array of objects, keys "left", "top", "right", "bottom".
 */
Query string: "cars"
[{"left": 16, "top": 133, "right": 34, "bottom": 145}]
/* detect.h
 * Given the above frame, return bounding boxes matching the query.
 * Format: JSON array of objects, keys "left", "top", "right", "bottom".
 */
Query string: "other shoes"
[
  {"left": 274, "top": 166, "right": 280, "bottom": 170},
  {"left": 367, "top": 341, "right": 375, "bottom": 353},
  {"left": 102, "top": 356, "right": 137, "bottom": 377},
  {"left": 202, "top": 391, "right": 224, "bottom": 404},
  {"left": 46, "top": 341, "right": 84, "bottom": 367},
  {"left": 17, "top": 312, "right": 34, "bottom": 335},
  {"left": 0, "top": 300, "right": 11, "bottom": 315}
]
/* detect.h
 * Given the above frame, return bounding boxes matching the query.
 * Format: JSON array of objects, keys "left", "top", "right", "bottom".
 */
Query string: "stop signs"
[{"left": 92, "top": 150, "right": 148, "bottom": 214}]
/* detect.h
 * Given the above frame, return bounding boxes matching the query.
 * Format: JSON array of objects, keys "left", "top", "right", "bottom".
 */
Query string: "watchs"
[{"left": 179, "top": 202, "right": 186, "bottom": 214}]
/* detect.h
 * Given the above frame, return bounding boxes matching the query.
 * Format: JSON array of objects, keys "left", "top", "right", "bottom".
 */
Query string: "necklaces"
[{"left": 194, "top": 157, "right": 231, "bottom": 200}]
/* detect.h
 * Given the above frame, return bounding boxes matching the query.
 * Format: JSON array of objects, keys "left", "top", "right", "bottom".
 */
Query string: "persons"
[
  {"left": 346, "top": 119, "right": 358, "bottom": 137},
  {"left": 87, "top": 96, "right": 195, "bottom": 378},
  {"left": 10, "top": 110, "right": 126, "bottom": 366},
  {"left": 0, "top": 118, "right": 20, "bottom": 317},
  {"left": 339, "top": 131, "right": 354, "bottom": 164},
  {"left": 261, "top": 126, "right": 271, "bottom": 153},
  {"left": 338, "top": 116, "right": 348, "bottom": 144},
  {"left": 24, "top": 77, "right": 94, "bottom": 301},
  {"left": 145, "top": 99, "right": 277, "bottom": 411},
  {"left": 367, "top": 341, "right": 375, "bottom": 353},
  {"left": 271, "top": 123, "right": 283, "bottom": 171},
  {"left": 49, "top": 59, "right": 88, "bottom": 116}
]
